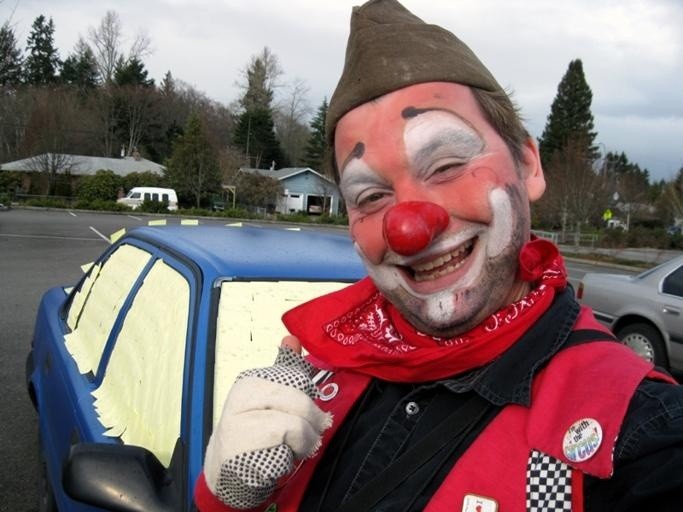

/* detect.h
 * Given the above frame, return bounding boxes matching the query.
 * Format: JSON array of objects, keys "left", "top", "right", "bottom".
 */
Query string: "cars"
[
  {"left": 667, "top": 225, "right": 681, "bottom": 236},
  {"left": 24, "top": 215, "right": 373, "bottom": 510},
  {"left": 211, "top": 200, "right": 228, "bottom": 213},
  {"left": 307, "top": 203, "right": 321, "bottom": 215},
  {"left": 577, "top": 254, "right": 682, "bottom": 384}
]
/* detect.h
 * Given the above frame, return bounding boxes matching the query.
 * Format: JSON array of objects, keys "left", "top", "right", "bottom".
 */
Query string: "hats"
[{"left": 324, "top": 0, "right": 515, "bottom": 151}]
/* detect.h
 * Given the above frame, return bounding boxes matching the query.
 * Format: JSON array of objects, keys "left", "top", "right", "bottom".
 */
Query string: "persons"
[{"left": 187, "top": 1, "right": 682, "bottom": 511}]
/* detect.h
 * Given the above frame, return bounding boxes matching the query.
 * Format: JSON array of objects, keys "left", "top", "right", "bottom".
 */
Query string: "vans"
[{"left": 116, "top": 187, "right": 181, "bottom": 215}]
